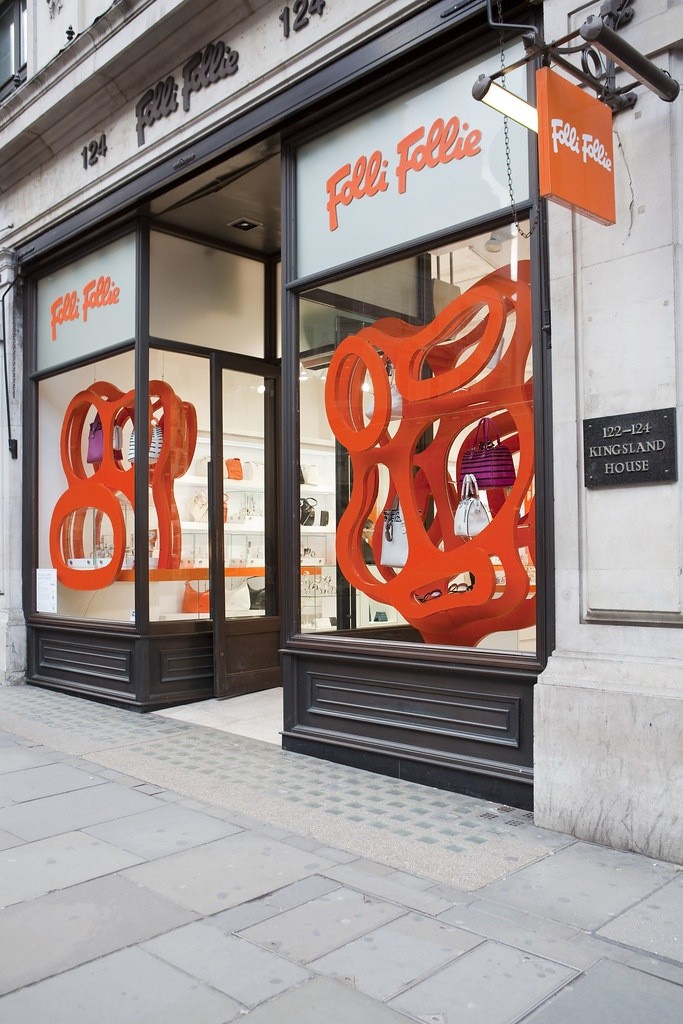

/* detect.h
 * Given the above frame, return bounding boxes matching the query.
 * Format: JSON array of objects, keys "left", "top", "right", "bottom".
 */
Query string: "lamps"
[
  {"left": 471, "top": 73, "right": 539, "bottom": 136},
  {"left": 579, "top": 13, "right": 679, "bottom": 103}
]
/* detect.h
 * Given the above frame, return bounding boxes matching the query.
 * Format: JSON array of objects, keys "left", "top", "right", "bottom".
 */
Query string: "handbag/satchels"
[
  {"left": 191, "top": 456, "right": 318, "bottom": 525},
  {"left": 454, "top": 474, "right": 490, "bottom": 537},
  {"left": 246, "top": 576, "right": 266, "bottom": 609},
  {"left": 380, "top": 493, "right": 410, "bottom": 568},
  {"left": 87, "top": 411, "right": 124, "bottom": 463},
  {"left": 459, "top": 418, "right": 516, "bottom": 488},
  {"left": 366, "top": 366, "right": 404, "bottom": 422},
  {"left": 129, "top": 426, "right": 163, "bottom": 464},
  {"left": 181, "top": 581, "right": 211, "bottom": 613}
]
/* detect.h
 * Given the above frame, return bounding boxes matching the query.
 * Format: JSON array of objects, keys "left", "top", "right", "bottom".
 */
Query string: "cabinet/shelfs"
[
  {"left": 46, "top": 382, "right": 198, "bottom": 592},
  {"left": 320, "top": 262, "right": 542, "bottom": 650},
  {"left": 106, "top": 413, "right": 406, "bottom": 634}
]
